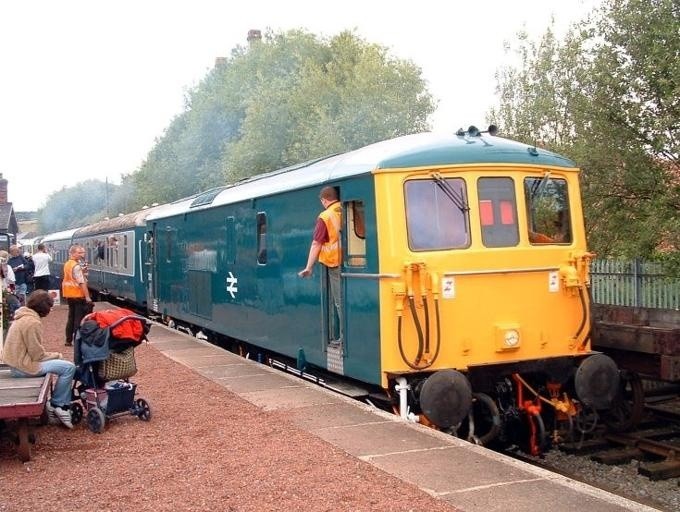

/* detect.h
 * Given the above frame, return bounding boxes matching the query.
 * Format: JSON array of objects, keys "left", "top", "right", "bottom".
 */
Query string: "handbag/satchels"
[{"left": 98, "top": 345, "right": 138, "bottom": 381}]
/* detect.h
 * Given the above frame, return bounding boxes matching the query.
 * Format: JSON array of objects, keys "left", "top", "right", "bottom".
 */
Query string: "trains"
[{"left": 20, "top": 125, "right": 646, "bottom": 448}]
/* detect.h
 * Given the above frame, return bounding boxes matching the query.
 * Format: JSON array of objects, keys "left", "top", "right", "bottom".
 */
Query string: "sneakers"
[
  {"left": 46, "top": 401, "right": 59, "bottom": 421},
  {"left": 53, "top": 406, "right": 73, "bottom": 429}
]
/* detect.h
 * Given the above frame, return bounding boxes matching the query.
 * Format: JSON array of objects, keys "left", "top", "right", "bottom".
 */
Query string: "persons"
[
  {"left": 531, "top": 208, "right": 569, "bottom": 243},
  {"left": 4, "top": 289, "right": 76, "bottom": 428},
  {"left": 0, "top": 244, "right": 52, "bottom": 316},
  {"left": 64, "top": 246, "right": 92, "bottom": 346},
  {"left": 296, "top": 186, "right": 344, "bottom": 344},
  {"left": 81, "top": 248, "right": 89, "bottom": 282}
]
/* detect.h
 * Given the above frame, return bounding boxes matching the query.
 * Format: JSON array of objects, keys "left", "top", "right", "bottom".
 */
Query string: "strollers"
[{"left": 70, "top": 309, "right": 150, "bottom": 432}]
